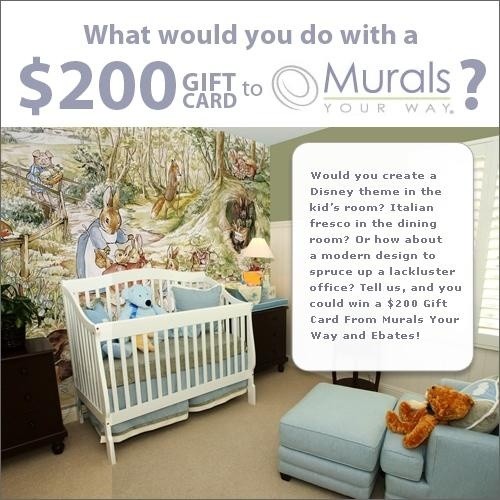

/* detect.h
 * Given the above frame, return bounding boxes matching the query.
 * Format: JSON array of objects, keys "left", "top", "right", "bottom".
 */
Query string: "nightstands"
[
  {"left": 0, "top": 337, "right": 68, "bottom": 464},
  {"left": 253, "top": 307, "right": 289, "bottom": 375}
]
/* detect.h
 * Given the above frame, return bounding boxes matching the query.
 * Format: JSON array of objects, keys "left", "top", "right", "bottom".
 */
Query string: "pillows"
[
  {"left": 85, "top": 285, "right": 223, "bottom": 358},
  {"left": 447, "top": 378, "right": 499, "bottom": 433}
]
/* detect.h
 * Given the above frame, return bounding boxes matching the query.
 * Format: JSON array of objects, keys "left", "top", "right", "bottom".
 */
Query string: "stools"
[{"left": 277, "top": 382, "right": 398, "bottom": 500}]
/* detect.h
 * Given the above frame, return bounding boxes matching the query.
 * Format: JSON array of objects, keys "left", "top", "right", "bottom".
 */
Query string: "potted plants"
[{"left": 0, "top": 279, "right": 47, "bottom": 359}]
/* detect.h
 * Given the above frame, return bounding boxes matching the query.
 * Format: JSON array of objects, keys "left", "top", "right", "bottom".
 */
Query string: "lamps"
[{"left": 242, "top": 237, "right": 274, "bottom": 259}]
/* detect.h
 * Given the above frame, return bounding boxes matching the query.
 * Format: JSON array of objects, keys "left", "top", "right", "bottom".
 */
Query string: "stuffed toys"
[{"left": 383, "top": 383, "right": 476, "bottom": 450}]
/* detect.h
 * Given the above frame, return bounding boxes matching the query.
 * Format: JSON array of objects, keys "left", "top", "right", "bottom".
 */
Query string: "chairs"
[{"left": 378, "top": 376, "right": 500, "bottom": 500}]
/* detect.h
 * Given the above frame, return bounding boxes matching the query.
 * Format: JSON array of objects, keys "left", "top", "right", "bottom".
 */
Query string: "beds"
[{"left": 61, "top": 269, "right": 258, "bottom": 466}]
